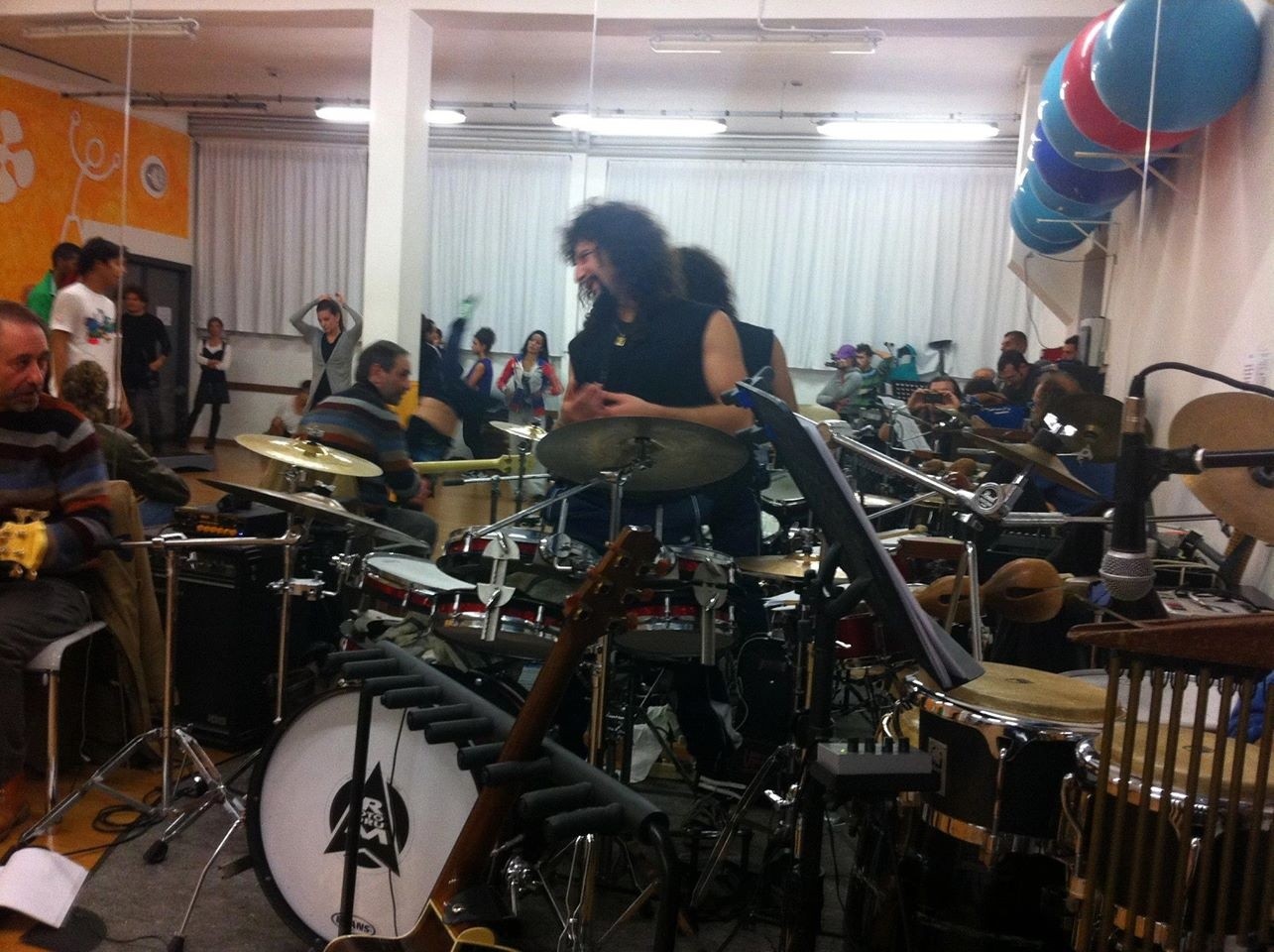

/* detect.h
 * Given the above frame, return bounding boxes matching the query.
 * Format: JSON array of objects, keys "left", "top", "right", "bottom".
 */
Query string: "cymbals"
[
  {"left": 236, "top": 432, "right": 386, "bottom": 480},
  {"left": 852, "top": 491, "right": 900, "bottom": 509},
  {"left": 911, "top": 493, "right": 969, "bottom": 512},
  {"left": 196, "top": 476, "right": 430, "bottom": 550},
  {"left": 490, "top": 420, "right": 552, "bottom": 444},
  {"left": 737, "top": 552, "right": 856, "bottom": 583},
  {"left": 964, "top": 427, "right": 1101, "bottom": 497},
  {"left": 535, "top": 414, "right": 749, "bottom": 496}
]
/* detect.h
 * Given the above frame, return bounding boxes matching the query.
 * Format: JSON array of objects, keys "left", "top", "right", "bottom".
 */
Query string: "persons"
[
  {"left": 460, "top": 326, "right": 493, "bottom": 442},
  {"left": 288, "top": 289, "right": 364, "bottom": 418},
  {"left": 537, "top": 194, "right": 758, "bottom": 604},
  {"left": 432, "top": 327, "right": 446, "bottom": 356},
  {"left": 418, "top": 312, "right": 441, "bottom": 408},
  {"left": 404, "top": 292, "right": 488, "bottom": 494},
  {"left": 264, "top": 377, "right": 314, "bottom": 438},
  {"left": 496, "top": 329, "right": 566, "bottom": 459},
  {"left": 117, "top": 280, "right": 173, "bottom": 461},
  {"left": 50, "top": 235, "right": 136, "bottom": 432},
  {"left": 26, "top": 239, "right": 84, "bottom": 343},
  {"left": 654, "top": 243, "right": 803, "bottom": 633},
  {"left": 811, "top": 323, "right": 1161, "bottom": 617},
  {"left": 297, "top": 338, "right": 442, "bottom": 562},
  {"left": 168, "top": 316, "right": 235, "bottom": 453},
  {"left": 0, "top": 294, "right": 112, "bottom": 851},
  {"left": 57, "top": 358, "right": 194, "bottom": 509}
]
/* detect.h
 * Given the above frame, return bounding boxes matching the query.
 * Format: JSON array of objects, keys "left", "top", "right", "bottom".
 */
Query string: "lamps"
[
  {"left": 552, "top": 110, "right": 725, "bottom": 140},
  {"left": 130, "top": 98, "right": 268, "bottom": 115},
  {"left": 649, "top": 28, "right": 886, "bottom": 56},
  {"left": 814, "top": 119, "right": 999, "bottom": 138},
  {"left": 315, "top": 100, "right": 466, "bottom": 126},
  {"left": 23, "top": 18, "right": 197, "bottom": 40}
]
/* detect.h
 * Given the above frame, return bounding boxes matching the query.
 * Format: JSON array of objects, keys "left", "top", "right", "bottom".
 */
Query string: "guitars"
[
  {"left": 318, "top": 527, "right": 652, "bottom": 949},
  {"left": 0, "top": 502, "right": 50, "bottom": 583}
]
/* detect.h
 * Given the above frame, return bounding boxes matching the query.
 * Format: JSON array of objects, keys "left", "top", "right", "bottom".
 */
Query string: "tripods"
[{"left": 14, "top": 520, "right": 304, "bottom": 860}]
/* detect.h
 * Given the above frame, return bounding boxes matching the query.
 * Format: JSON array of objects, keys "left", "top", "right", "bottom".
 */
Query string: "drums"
[
  {"left": 901, "top": 659, "right": 1126, "bottom": 862},
  {"left": 644, "top": 545, "right": 733, "bottom": 583},
  {"left": 361, "top": 554, "right": 477, "bottom": 613},
  {"left": 759, "top": 512, "right": 780, "bottom": 546},
  {"left": 251, "top": 671, "right": 481, "bottom": 943},
  {"left": 448, "top": 525, "right": 570, "bottom": 569},
  {"left": 1062, "top": 723, "right": 1273, "bottom": 949},
  {"left": 761, "top": 471, "right": 808, "bottom": 519},
  {"left": 613, "top": 603, "right": 736, "bottom": 662},
  {"left": 427, "top": 597, "right": 561, "bottom": 662}
]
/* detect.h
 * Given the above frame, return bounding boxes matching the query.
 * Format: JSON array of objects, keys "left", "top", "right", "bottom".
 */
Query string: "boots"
[{"left": 207, "top": 415, "right": 222, "bottom": 445}]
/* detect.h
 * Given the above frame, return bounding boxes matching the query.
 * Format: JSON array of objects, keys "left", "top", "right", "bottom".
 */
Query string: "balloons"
[{"left": 1007, "top": 0, "right": 1262, "bottom": 256}]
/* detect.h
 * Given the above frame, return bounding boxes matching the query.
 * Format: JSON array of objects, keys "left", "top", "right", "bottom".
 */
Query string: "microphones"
[{"left": 1091, "top": 363, "right": 1164, "bottom": 597}]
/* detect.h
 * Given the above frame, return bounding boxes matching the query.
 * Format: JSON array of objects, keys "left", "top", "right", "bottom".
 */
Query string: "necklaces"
[{"left": 613, "top": 326, "right": 633, "bottom": 349}]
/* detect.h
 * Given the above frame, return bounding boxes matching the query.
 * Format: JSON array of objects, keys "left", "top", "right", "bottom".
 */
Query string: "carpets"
[{"left": 67, "top": 757, "right": 318, "bottom": 951}]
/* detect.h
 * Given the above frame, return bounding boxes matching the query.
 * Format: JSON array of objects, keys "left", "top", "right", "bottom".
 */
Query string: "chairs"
[{"left": 23, "top": 480, "right": 163, "bottom": 825}]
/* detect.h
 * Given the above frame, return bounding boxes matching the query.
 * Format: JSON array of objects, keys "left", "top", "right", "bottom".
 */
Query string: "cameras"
[{"left": 923, "top": 390, "right": 943, "bottom": 403}]
